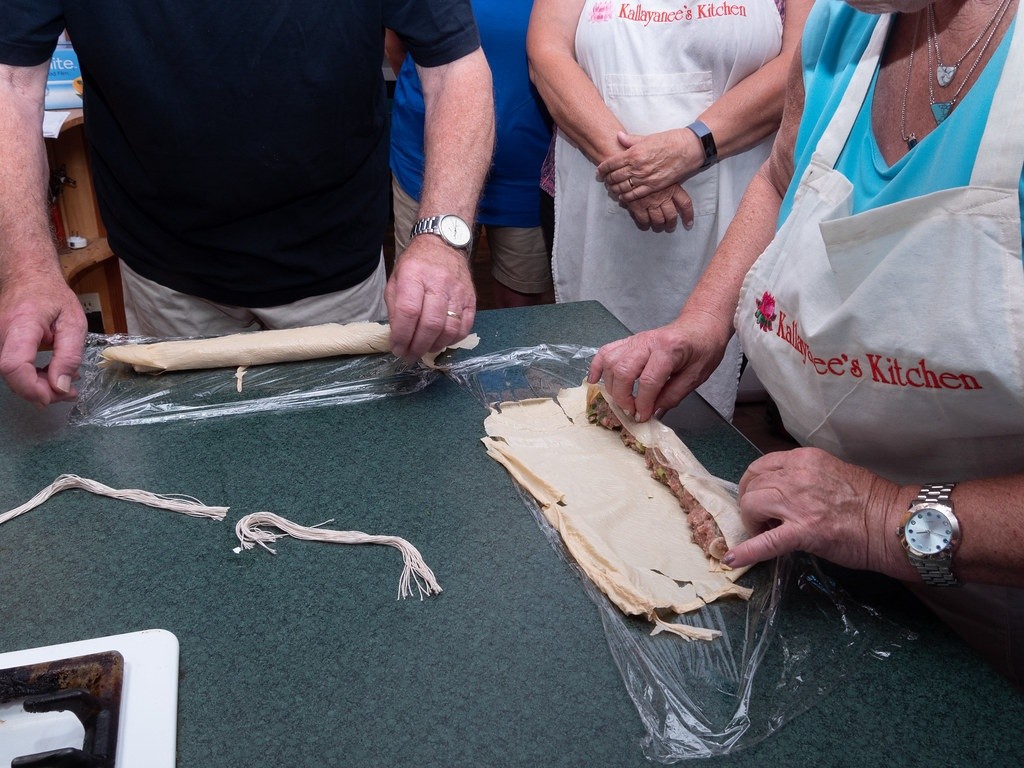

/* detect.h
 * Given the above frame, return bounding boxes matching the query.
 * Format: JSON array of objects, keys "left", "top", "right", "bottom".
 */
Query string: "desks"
[{"left": 1, "top": 298, "right": 1024, "bottom": 768}]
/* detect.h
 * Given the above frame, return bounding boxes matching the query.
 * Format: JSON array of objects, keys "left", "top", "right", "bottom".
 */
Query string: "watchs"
[
  {"left": 410, "top": 213, "right": 474, "bottom": 259},
  {"left": 895, "top": 482, "right": 961, "bottom": 589},
  {"left": 686, "top": 120, "right": 719, "bottom": 168}
]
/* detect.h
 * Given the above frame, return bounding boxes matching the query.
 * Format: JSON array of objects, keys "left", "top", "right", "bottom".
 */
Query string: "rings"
[
  {"left": 629, "top": 178, "right": 634, "bottom": 187},
  {"left": 447, "top": 310, "right": 462, "bottom": 319}
]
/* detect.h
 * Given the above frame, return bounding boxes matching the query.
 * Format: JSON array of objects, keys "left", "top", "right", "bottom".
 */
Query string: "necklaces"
[{"left": 900, "top": 0, "right": 1011, "bottom": 151}]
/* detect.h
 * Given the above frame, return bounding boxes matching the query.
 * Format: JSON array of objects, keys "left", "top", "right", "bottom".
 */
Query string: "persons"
[
  {"left": 525, "top": 0, "right": 821, "bottom": 421},
  {"left": 384, "top": 0, "right": 552, "bottom": 308},
  {"left": 0, "top": 0, "right": 499, "bottom": 407},
  {"left": 586, "top": 0, "right": 1024, "bottom": 699}
]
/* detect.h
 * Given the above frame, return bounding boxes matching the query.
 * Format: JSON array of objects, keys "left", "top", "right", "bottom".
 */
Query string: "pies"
[
  {"left": 96, "top": 319, "right": 482, "bottom": 373},
  {"left": 481, "top": 377, "right": 767, "bottom": 638}
]
